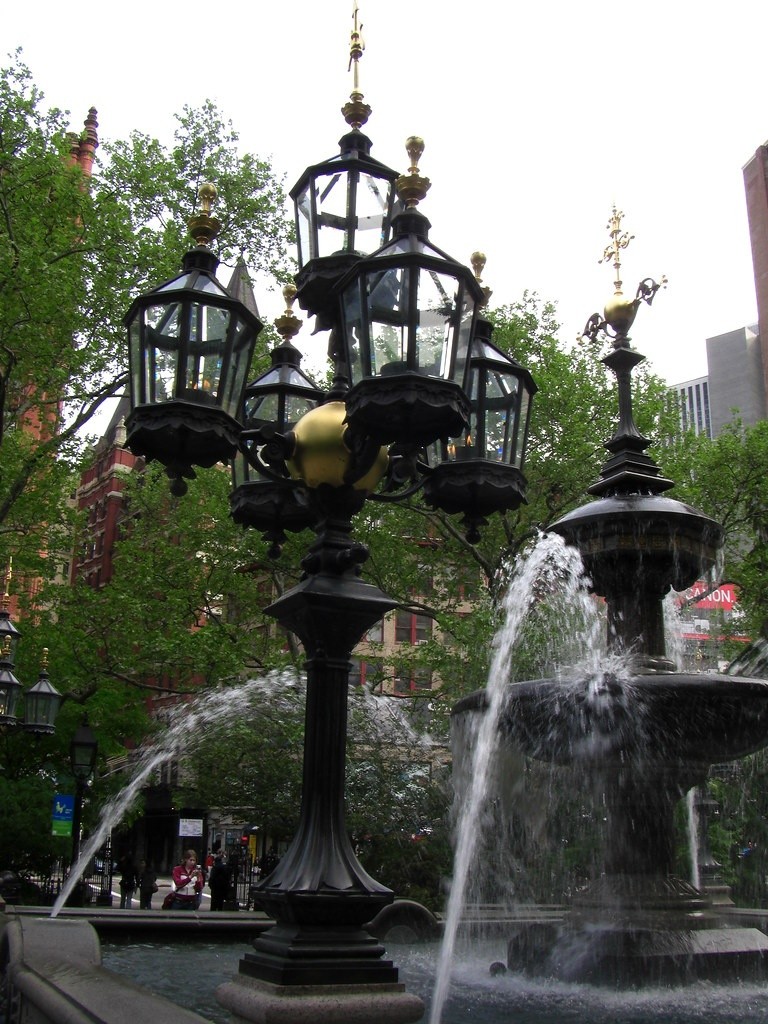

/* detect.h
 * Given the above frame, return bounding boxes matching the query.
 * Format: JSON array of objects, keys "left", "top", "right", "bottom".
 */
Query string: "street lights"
[
  {"left": 125, "top": 0, "right": 533, "bottom": 992},
  {"left": 59, "top": 710, "right": 100, "bottom": 907}
]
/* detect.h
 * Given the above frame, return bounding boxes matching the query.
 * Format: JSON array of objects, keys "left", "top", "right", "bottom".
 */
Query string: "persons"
[
  {"left": 169, "top": 849, "right": 226, "bottom": 911},
  {"left": 119, "top": 859, "right": 157, "bottom": 909}
]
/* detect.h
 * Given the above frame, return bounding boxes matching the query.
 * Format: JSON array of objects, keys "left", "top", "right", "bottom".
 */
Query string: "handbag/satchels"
[
  {"left": 152, "top": 882, "right": 158, "bottom": 892},
  {"left": 119, "top": 880, "right": 135, "bottom": 892},
  {"left": 162, "top": 893, "right": 174, "bottom": 909}
]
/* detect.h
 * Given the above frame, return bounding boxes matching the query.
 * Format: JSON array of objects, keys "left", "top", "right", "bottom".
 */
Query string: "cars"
[
  {"left": 65, "top": 881, "right": 95, "bottom": 908},
  {"left": 0, "top": 871, "right": 40, "bottom": 906}
]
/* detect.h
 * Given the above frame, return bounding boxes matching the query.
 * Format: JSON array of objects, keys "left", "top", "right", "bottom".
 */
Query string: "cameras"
[{"left": 196, "top": 865, "right": 201, "bottom": 871}]
